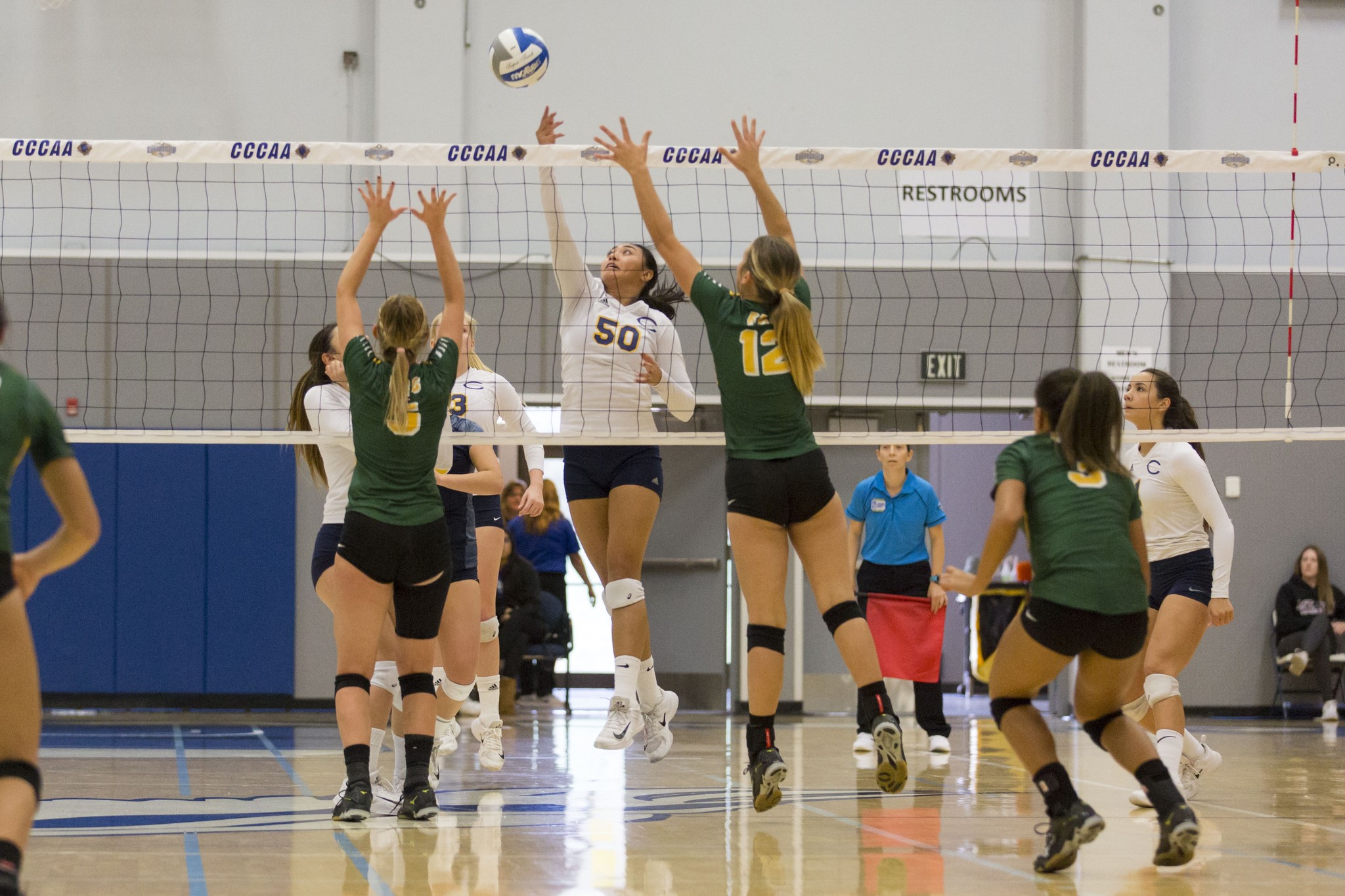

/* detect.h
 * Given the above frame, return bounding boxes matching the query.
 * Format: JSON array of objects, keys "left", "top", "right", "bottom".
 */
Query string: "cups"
[{"left": 1017, "top": 560, "right": 1033, "bottom": 582}]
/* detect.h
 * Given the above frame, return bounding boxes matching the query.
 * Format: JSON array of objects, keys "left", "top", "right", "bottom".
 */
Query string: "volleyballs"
[{"left": 486, "top": 26, "right": 550, "bottom": 90}]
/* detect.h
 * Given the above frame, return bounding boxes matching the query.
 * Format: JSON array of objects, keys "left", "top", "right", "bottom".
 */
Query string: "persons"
[
  {"left": 1273, "top": 545, "right": 1344, "bottom": 721},
  {"left": 0, "top": 299, "right": 100, "bottom": 896},
  {"left": 846, "top": 428, "right": 951, "bottom": 753},
  {"left": 935, "top": 366, "right": 1199, "bottom": 874},
  {"left": 1118, "top": 368, "right": 1235, "bottom": 809},
  {"left": 594, "top": 114, "right": 908, "bottom": 813},
  {"left": 533, "top": 106, "right": 697, "bottom": 765},
  {"left": 286, "top": 176, "right": 597, "bottom": 820}
]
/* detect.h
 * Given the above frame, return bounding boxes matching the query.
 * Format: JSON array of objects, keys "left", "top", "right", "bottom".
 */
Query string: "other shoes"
[
  {"left": 875, "top": 855, "right": 908, "bottom": 894},
  {"left": 1288, "top": 650, "right": 1309, "bottom": 676},
  {"left": 1322, "top": 720, "right": 1340, "bottom": 742},
  {"left": 929, "top": 734, "right": 951, "bottom": 753},
  {"left": 334, "top": 816, "right": 463, "bottom": 856},
  {"left": 748, "top": 832, "right": 788, "bottom": 892},
  {"left": 852, "top": 732, "right": 875, "bottom": 751},
  {"left": 1036, "top": 870, "right": 1080, "bottom": 896},
  {"left": 516, "top": 692, "right": 565, "bottom": 708},
  {"left": 852, "top": 752, "right": 876, "bottom": 769},
  {"left": 472, "top": 792, "right": 505, "bottom": 853},
  {"left": 1320, "top": 698, "right": 1339, "bottom": 720},
  {"left": 927, "top": 752, "right": 952, "bottom": 768}
]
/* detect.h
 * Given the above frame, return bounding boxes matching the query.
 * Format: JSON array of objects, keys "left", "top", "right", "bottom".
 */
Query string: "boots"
[{"left": 497, "top": 673, "right": 518, "bottom": 717}]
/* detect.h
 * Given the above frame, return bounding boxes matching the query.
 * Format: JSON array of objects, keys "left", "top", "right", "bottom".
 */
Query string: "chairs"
[
  {"left": 1272, "top": 609, "right": 1345, "bottom": 722},
  {"left": 512, "top": 613, "right": 572, "bottom": 716}
]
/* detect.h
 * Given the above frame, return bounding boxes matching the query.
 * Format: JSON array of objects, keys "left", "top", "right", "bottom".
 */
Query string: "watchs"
[{"left": 929, "top": 574, "right": 940, "bottom": 583}]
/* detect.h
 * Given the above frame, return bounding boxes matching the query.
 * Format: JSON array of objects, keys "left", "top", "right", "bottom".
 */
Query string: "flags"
[{"left": 866, "top": 591, "right": 946, "bottom": 684}]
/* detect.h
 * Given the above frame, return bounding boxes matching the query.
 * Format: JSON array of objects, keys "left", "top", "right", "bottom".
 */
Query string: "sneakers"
[
  {"left": 427, "top": 740, "right": 442, "bottom": 789},
  {"left": 471, "top": 714, "right": 504, "bottom": 771},
  {"left": 332, "top": 785, "right": 371, "bottom": 821},
  {"left": 639, "top": 686, "right": 679, "bottom": 764},
  {"left": 459, "top": 696, "right": 483, "bottom": 715},
  {"left": 1130, "top": 782, "right": 1188, "bottom": 806},
  {"left": 870, "top": 713, "right": 908, "bottom": 793},
  {"left": 1151, "top": 802, "right": 1200, "bottom": 866},
  {"left": 388, "top": 787, "right": 440, "bottom": 819},
  {"left": 742, "top": 746, "right": 788, "bottom": 813},
  {"left": 594, "top": 697, "right": 646, "bottom": 751},
  {"left": 1179, "top": 733, "right": 1222, "bottom": 800},
  {"left": 1033, "top": 803, "right": 1106, "bottom": 874},
  {"left": 333, "top": 769, "right": 404, "bottom": 818},
  {"left": 437, "top": 716, "right": 462, "bottom": 754}
]
